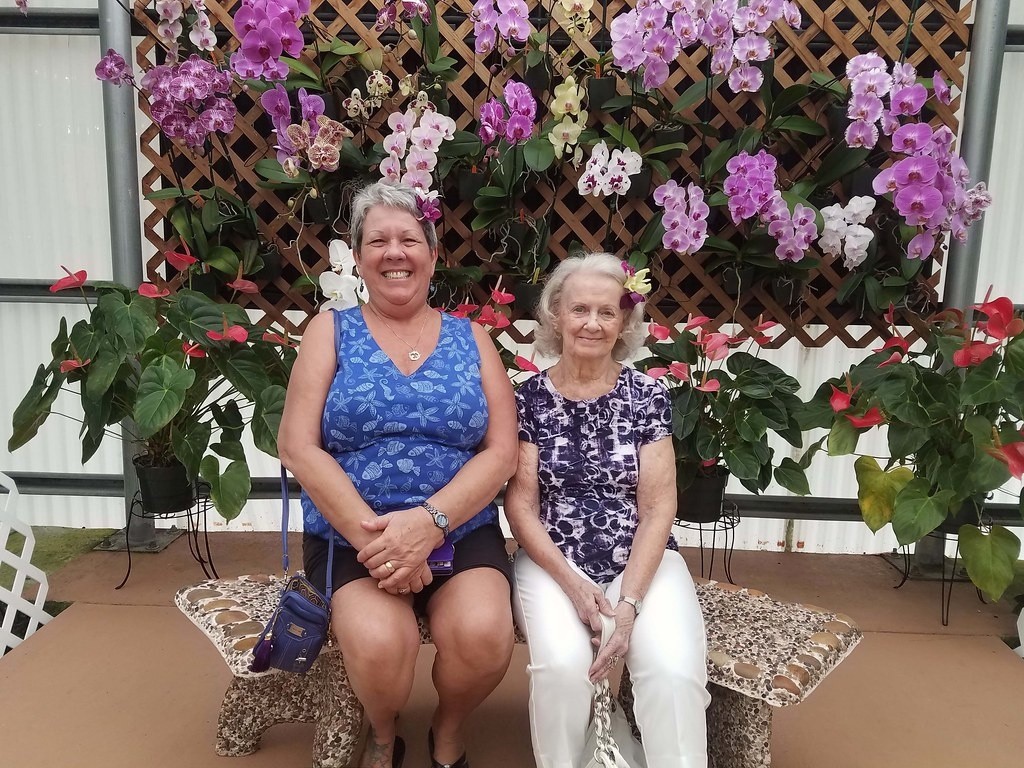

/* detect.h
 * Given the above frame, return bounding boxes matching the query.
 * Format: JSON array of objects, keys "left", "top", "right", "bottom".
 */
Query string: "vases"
[
  {"left": 928, "top": 481, "right": 990, "bottom": 530},
  {"left": 135, "top": 451, "right": 198, "bottom": 514},
  {"left": 677, "top": 463, "right": 731, "bottom": 524}
]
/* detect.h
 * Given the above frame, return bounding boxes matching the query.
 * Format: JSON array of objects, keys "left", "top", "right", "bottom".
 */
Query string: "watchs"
[
  {"left": 618, "top": 596, "right": 642, "bottom": 615},
  {"left": 420, "top": 501, "right": 450, "bottom": 538}
]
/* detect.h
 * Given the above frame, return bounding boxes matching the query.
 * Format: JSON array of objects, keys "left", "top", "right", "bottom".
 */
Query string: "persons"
[
  {"left": 278, "top": 182, "right": 519, "bottom": 768},
  {"left": 502, "top": 252, "right": 711, "bottom": 768}
]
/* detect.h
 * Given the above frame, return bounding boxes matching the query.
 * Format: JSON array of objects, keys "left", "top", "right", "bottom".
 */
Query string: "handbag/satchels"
[
  {"left": 580, "top": 612, "right": 647, "bottom": 768},
  {"left": 249, "top": 576, "right": 331, "bottom": 673}
]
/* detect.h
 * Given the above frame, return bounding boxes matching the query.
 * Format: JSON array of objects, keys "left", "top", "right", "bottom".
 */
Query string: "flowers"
[{"left": 8, "top": 0, "right": 1024, "bottom": 605}]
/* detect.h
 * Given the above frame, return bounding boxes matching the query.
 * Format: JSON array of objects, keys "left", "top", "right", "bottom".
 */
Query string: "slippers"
[
  {"left": 428, "top": 727, "right": 469, "bottom": 768},
  {"left": 392, "top": 735, "right": 405, "bottom": 768}
]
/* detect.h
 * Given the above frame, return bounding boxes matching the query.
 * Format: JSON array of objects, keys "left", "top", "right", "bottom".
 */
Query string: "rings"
[
  {"left": 385, "top": 561, "right": 395, "bottom": 575},
  {"left": 398, "top": 587, "right": 409, "bottom": 594},
  {"left": 613, "top": 655, "right": 619, "bottom": 663}
]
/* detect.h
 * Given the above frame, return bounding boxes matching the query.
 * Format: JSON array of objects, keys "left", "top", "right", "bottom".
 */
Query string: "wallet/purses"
[{"left": 427, "top": 541, "right": 455, "bottom": 575}]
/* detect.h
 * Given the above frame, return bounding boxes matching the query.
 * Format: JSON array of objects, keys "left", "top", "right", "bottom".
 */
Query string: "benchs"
[{"left": 173, "top": 568, "right": 866, "bottom": 767}]
